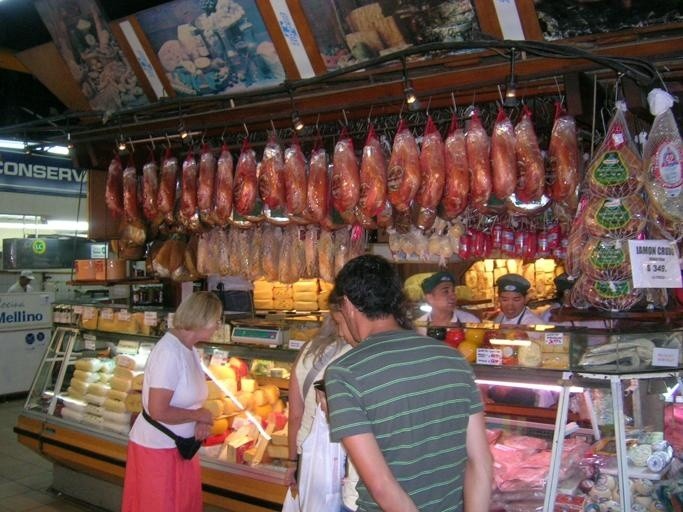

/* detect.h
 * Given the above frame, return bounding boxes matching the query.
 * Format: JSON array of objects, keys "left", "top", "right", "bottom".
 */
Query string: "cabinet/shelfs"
[
  {"left": 10, "top": 322, "right": 303, "bottom": 512},
  {"left": 339, "top": 324, "right": 683, "bottom": 512}
]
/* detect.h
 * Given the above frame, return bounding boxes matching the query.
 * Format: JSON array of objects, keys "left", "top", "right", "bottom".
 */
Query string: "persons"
[
  {"left": 413, "top": 271, "right": 480, "bottom": 337},
  {"left": 543, "top": 272, "right": 613, "bottom": 346},
  {"left": 481, "top": 274, "right": 561, "bottom": 412},
  {"left": 323, "top": 255, "right": 492, "bottom": 510},
  {"left": 283, "top": 287, "right": 363, "bottom": 510},
  {"left": 120, "top": 290, "right": 224, "bottom": 512},
  {"left": 6, "top": 270, "right": 35, "bottom": 294}
]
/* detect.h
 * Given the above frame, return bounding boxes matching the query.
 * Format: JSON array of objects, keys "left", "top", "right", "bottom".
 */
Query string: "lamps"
[{"left": 63, "top": 40, "right": 650, "bottom": 151}]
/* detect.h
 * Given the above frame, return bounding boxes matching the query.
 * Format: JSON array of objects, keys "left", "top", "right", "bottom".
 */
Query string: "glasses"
[{"left": 63, "top": 40, "right": 650, "bottom": 151}]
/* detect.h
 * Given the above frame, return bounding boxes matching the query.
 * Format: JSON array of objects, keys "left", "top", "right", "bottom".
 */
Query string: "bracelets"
[{"left": 288, "top": 459, "right": 298, "bottom": 462}]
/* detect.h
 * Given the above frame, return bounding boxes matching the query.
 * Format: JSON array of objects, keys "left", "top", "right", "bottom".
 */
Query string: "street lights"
[{"left": 177, "top": 436, "right": 201, "bottom": 459}]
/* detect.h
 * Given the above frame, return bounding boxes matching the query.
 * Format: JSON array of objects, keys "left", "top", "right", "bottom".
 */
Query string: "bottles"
[
  {"left": 421, "top": 271, "right": 455, "bottom": 293},
  {"left": 497, "top": 274, "right": 530, "bottom": 296},
  {"left": 555, "top": 272, "right": 571, "bottom": 291}
]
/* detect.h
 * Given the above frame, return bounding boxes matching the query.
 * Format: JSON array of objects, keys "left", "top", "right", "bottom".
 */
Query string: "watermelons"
[{"left": 230, "top": 318, "right": 287, "bottom": 349}]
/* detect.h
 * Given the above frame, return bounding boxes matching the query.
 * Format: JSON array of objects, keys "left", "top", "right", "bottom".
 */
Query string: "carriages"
[
  {"left": 62, "top": 350, "right": 289, "bottom": 466},
  {"left": 81, "top": 304, "right": 150, "bottom": 336},
  {"left": 464, "top": 258, "right": 565, "bottom": 308},
  {"left": 252, "top": 278, "right": 329, "bottom": 311}
]
